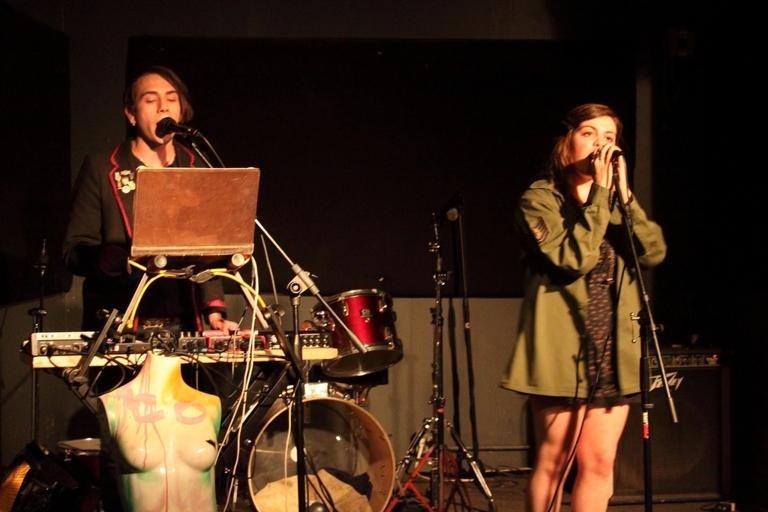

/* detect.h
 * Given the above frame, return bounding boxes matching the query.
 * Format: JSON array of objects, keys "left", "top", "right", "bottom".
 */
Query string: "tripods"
[{"left": 384, "top": 236, "right": 496, "bottom": 512}]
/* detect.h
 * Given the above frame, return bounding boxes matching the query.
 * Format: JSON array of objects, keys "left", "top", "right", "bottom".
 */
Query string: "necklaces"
[{"left": 131, "top": 142, "right": 176, "bottom": 169}]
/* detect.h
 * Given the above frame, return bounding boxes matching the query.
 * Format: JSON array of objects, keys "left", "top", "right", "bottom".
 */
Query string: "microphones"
[
  {"left": 155, "top": 118, "right": 203, "bottom": 143},
  {"left": 592, "top": 149, "right": 628, "bottom": 170},
  {"left": 444, "top": 192, "right": 465, "bottom": 223}
]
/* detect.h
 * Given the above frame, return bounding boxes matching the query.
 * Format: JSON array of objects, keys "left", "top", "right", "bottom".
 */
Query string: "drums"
[
  {"left": 310, "top": 287, "right": 403, "bottom": 377},
  {"left": 59, "top": 439, "right": 100, "bottom": 466},
  {"left": 218, "top": 392, "right": 397, "bottom": 511}
]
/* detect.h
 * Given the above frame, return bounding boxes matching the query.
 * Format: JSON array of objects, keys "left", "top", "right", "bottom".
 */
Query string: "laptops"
[{"left": 135, "top": 166, "right": 262, "bottom": 257}]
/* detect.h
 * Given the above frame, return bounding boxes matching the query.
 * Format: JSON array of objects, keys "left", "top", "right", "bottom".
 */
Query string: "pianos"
[{"left": 21, "top": 330, "right": 339, "bottom": 369}]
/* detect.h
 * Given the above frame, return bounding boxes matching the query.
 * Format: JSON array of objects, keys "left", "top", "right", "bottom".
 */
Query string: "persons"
[
  {"left": 56, "top": 56, "right": 238, "bottom": 512},
  {"left": 493, "top": 97, "right": 670, "bottom": 509},
  {"left": 102, "top": 356, "right": 228, "bottom": 511}
]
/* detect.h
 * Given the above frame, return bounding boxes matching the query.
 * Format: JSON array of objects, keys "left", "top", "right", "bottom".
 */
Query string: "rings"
[{"left": 594, "top": 152, "right": 600, "bottom": 160}]
